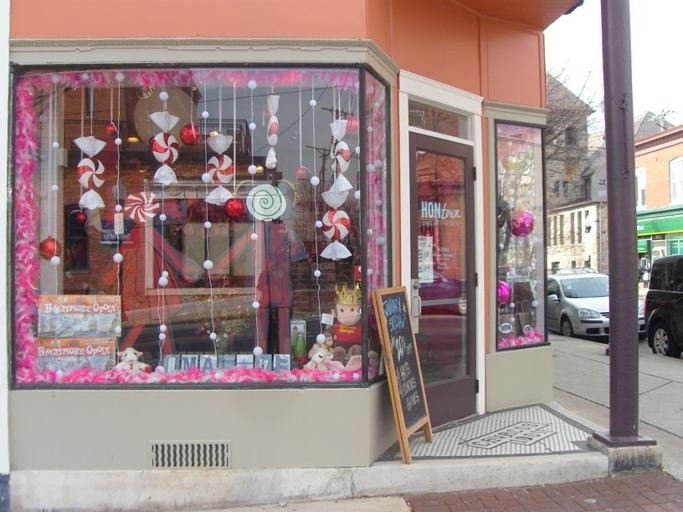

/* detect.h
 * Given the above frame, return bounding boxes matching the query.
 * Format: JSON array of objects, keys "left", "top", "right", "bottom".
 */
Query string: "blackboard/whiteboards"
[{"left": 372, "top": 287, "right": 429, "bottom": 437}]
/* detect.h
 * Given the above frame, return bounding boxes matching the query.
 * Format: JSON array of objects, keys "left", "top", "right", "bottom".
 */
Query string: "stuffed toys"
[
  {"left": 113, "top": 347, "right": 151, "bottom": 379},
  {"left": 303, "top": 283, "right": 379, "bottom": 376}
]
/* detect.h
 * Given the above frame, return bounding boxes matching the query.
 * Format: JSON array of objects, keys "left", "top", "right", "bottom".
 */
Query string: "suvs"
[{"left": 643, "top": 259, "right": 682, "bottom": 356}]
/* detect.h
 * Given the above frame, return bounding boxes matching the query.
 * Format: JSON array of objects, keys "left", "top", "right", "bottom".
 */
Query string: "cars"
[
  {"left": 545, "top": 272, "right": 648, "bottom": 343},
  {"left": 416, "top": 269, "right": 462, "bottom": 315}
]
